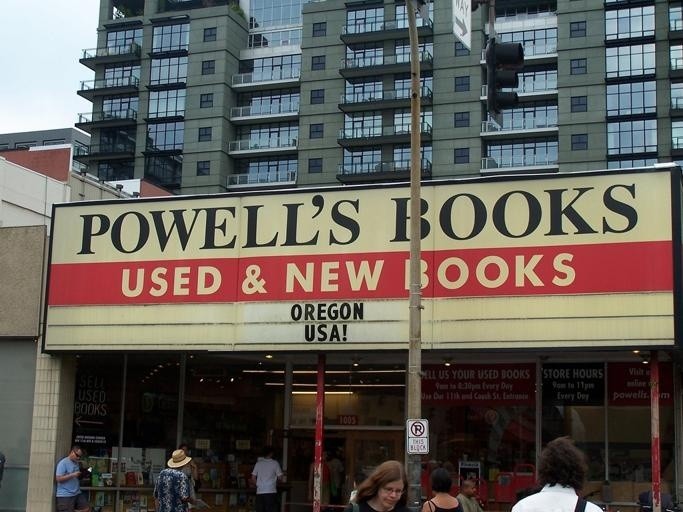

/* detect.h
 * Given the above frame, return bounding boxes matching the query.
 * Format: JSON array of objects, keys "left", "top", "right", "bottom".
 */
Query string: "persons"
[
  {"left": 510, "top": 434, "right": 605, "bottom": 511},
  {"left": 308, "top": 451, "right": 334, "bottom": 504},
  {"left": 349, "top": 471, "right": 362, "bottom": 501},
  {"left": 455, "top": 479, "right": 485, "bottom": 511},
  {"left": 181, "top": 442, "right": 199, "bottom": 512},
  {"left": 325, "top": 447, "right": 344, "bottom": 504},
  {"left": 54, "top": 444, "right": 90, "bottom": 512},
  {"left": 249, "top": 445, "right": 284, "bottom": 511},
  {"left": 342, "top": 459, "right": 411, "bottom": 511},
  {"left": 151, "top": 448, "right": 202, "bottom": 512},
  {"left": 422, "top": 466, "right": 463, "bottom": 511}
]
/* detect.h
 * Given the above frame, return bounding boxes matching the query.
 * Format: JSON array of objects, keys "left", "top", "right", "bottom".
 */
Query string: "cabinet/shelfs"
[{"left": 72, "top": 458, "right": 277, "bottom": 512}]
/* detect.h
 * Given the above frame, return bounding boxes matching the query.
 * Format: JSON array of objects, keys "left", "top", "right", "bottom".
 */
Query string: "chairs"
[{"left": 422, "top": 462, "right": 672, "bottom": 512}]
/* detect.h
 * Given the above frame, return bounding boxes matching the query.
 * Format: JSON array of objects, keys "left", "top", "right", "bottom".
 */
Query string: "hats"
[{"left": 167, "top": 449, "right": 192, "bottom": 468}]
